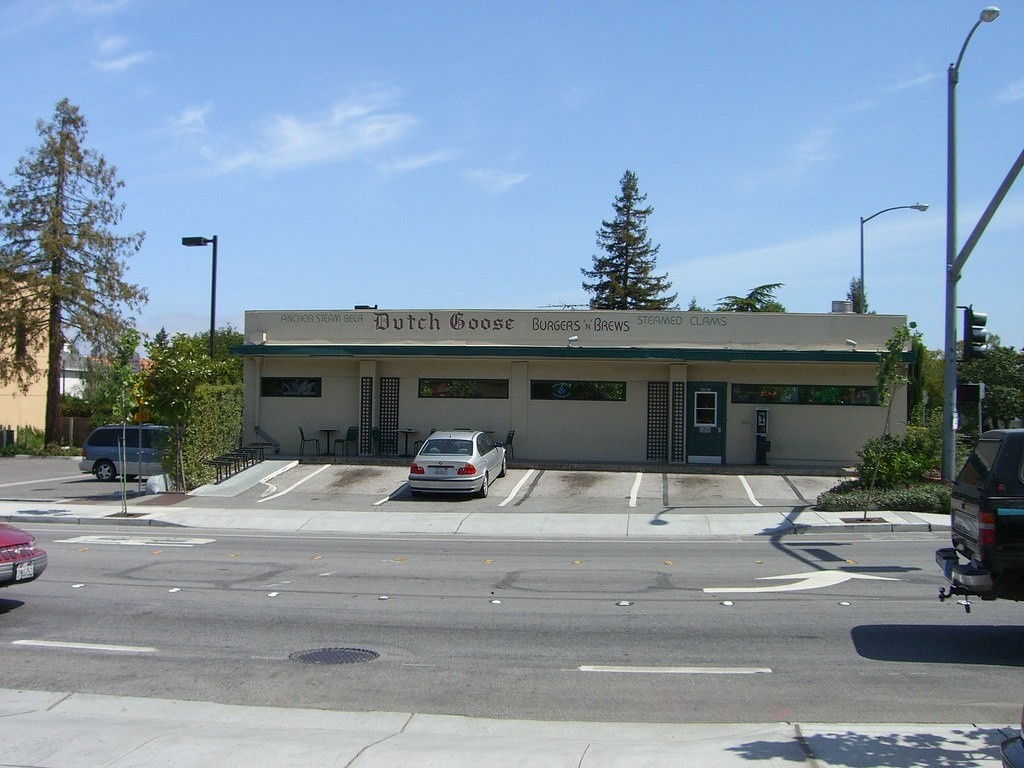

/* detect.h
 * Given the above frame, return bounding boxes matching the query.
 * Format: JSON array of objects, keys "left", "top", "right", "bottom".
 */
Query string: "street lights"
[
  {"left": 935, "top": 3, "right": 1020, "bottom": 487},
  {"left": 180, "top": 233, "right": 220, "bottom": 357},
  {"left": 855, "top": 199, "right": 930, "bottom": 305}
]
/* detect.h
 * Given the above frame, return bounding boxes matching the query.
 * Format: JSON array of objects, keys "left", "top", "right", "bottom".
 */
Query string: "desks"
[
  {"left": 316, "top": 428, "right": 339, "bottom": 455},
  {"left": 395, "top": 430, "right": 418, "bottom": 457},
  {"left": 481, "top": 431, "right": 496, "bottom": 433}
]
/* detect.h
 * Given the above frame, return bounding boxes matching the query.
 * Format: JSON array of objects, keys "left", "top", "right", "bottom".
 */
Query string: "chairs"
[
  {"left": 414, "top": 429, "right": 436, "bottom": 457},
  {"left": 496, "top": 430, "right": 515, "bottom": 461},
  {"left": 372, "top": 427, "right": 393, "bottom": 455},
  {"left": 334, "top": 426, "right": 359, "bottom": 455},
  {"left": 298, "top": 427, "right": 321, "bottom": 455}
]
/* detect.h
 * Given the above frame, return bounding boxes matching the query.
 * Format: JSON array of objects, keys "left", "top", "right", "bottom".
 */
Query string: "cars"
[
  {"left": 932, "top": 425, "right": 1022, "bottom": 613},
  {"left": 0, "top": 523, "right": 47, "bottom": 582},
  {"left": 406, "top": 429, "right": 509, "bottom": 501}
]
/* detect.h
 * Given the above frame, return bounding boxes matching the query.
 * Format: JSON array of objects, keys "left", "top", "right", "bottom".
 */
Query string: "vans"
[{"left": 75, "top": 426, "right": 174, "bottom": 482}]
